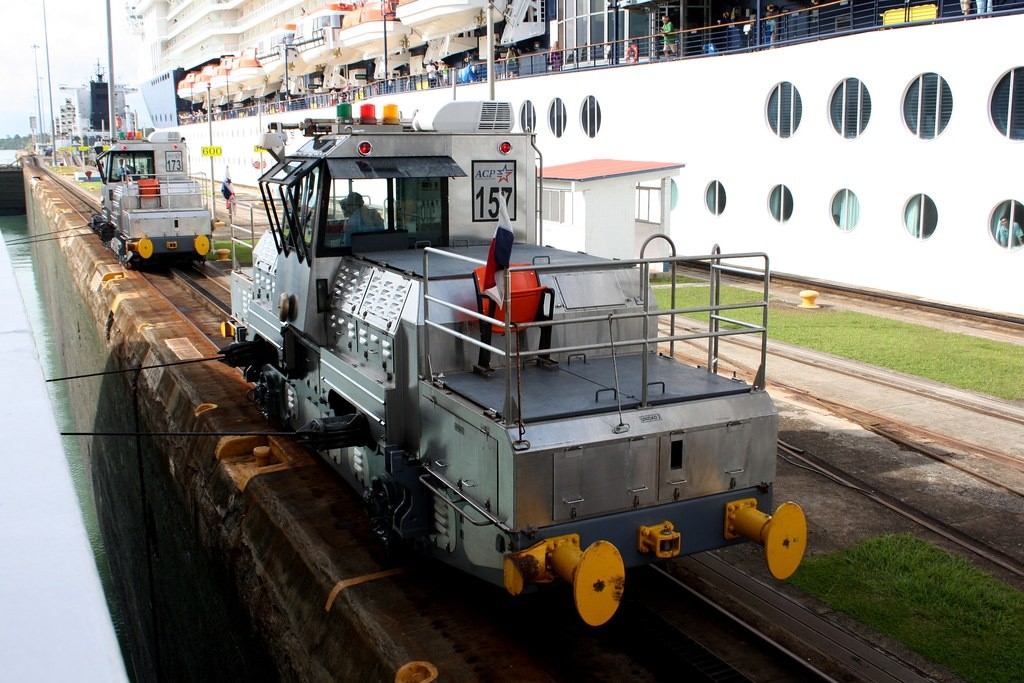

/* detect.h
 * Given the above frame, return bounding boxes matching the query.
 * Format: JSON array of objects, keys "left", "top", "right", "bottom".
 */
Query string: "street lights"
[{"left": 30, "top": 44, "right": 48, "bottom": 151}]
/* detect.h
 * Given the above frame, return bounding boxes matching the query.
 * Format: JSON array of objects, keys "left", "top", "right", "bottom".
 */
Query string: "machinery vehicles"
[
  {"left": 218, "top": 99, "right": 806, "bottom": 627},
  {"left": 87, "top": 131, "right": 212, "bottom": 270}
]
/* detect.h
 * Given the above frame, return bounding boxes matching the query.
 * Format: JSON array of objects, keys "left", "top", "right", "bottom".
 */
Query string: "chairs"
[
  {"left": 472, "top": 263, "right": 555, "bottom": 371},
  {"left": 138, "top": 178, "right": 161, "bottom": 208}
]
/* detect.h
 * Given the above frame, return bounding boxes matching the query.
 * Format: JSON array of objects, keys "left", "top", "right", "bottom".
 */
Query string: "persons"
[
  {"left": 339, "top": 191, "right": 385, "bottom": 245},
  {"left": 661, "top": 16, "right": 681, "bottom": 60},
  {"left": 118, "top": 157, "right": 140, "bottom": 181},
  {"left": 627, "top": 39, "right": 639, "bottom": 65},
  {"left": 176, "top": 41, "right": 564, "bottom": 126},
  {"left": 223, "top": 174, "right": 237, "bottom": 222},
  {"left": 723, "top": 0, "right": 822, "bottom": 55}
]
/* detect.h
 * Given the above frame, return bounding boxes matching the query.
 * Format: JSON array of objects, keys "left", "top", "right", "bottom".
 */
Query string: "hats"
[
  {"left": 427, "top": 59, "right": 435, "bottom": 64},
  {"left": 436, "top": 59, "right": 445, "bottom": 64},
  {"left": 226, "top": 178, "right": 232, "bottom": 185},
  {"left": 339, "top": 192, "right": 364, "bottom": 205}
]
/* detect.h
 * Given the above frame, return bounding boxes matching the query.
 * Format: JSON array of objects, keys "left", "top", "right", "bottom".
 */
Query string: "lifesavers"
[{"left": 625, "top": 45, "right": 639, "bottom": 64}]
